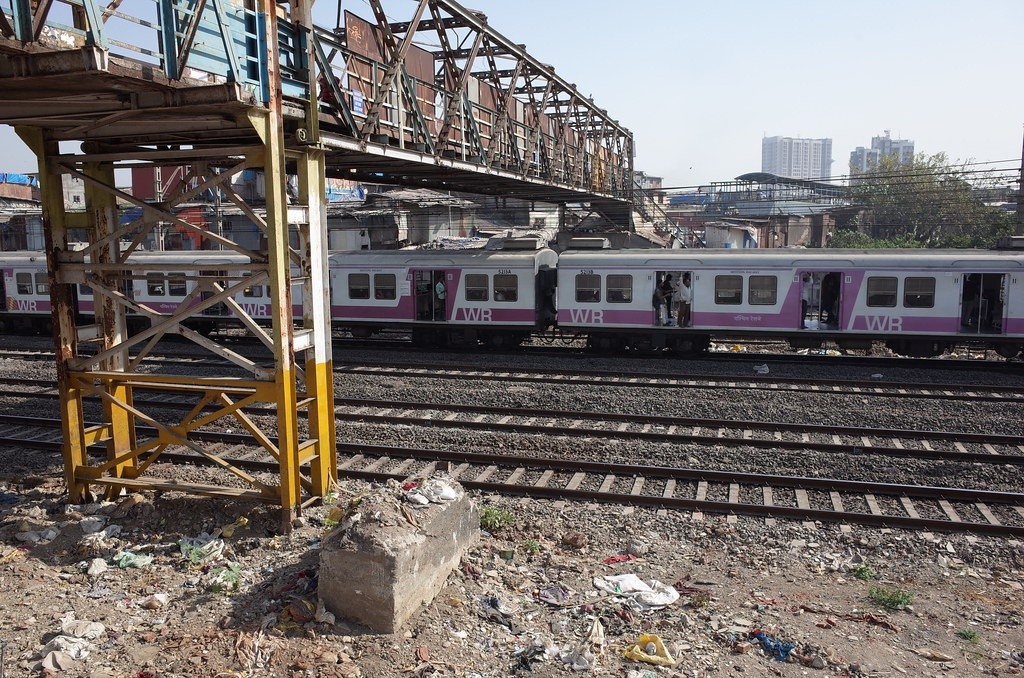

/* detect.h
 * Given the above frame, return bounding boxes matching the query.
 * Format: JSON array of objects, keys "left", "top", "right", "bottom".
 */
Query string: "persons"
[
  {"left": 676, "top": 272, "right": 691, "bottom": 326},
  {"left": 961, "top": 275, "right": 980, "bottom": 327},
  {"left": 824, "top": 273, "right": 841, "bottom": 323},
  {"left": 678, "top": 279, "right": 692, "bottom": 328},
  {"left": 655, "top": 279, "right": 674, "bottom": 327},
  {"left": 350, "top": 286, "right": 394, "bottom": 299},
  {"left": 662, "top": 274, "right": 678, "bottom": 319},
  {"left": 468, "top": 287, "right": 519, "bottom": 302},
  {"left": 146, "top": 228, "right": 187, "bottom": 295},
  {"left": 576, "top": 288, "right": 632, "bottom": 303},
  {"left": 819, "top": 273, "right": 832, "bottom": 322},
  {"left": 802, "top": 272, "right": 814, "bottom": 329},
  {"left": 435, "top": 274, "right": 449, "bottom": 321}
]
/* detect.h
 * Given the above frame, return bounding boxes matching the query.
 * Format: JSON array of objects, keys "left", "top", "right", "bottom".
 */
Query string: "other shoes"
[
  {"left": 663, "top": 321, "right": 671, "bottom": 326},
  {"left": 819, "top": 315, "right": 831, "bottom": 323},
  {"left": 802, "top": 326, "right": 808, "bottom": 329},
  {"left": 679, "top": 324, "right": 690, "bottom": 328},
  {"left": 669, "top": 316, "right": 675, "bottom": 318}
]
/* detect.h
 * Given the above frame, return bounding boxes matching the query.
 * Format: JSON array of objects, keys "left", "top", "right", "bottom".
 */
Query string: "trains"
[{"left": 2, "top": 248, "right": 1024, "bottom": 367}]
